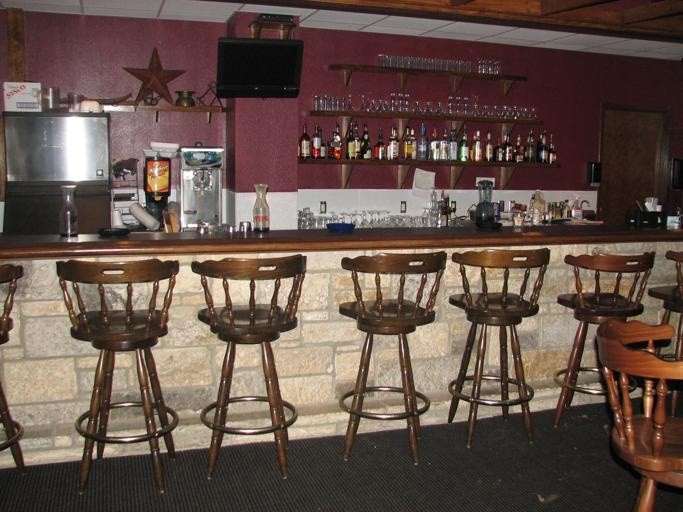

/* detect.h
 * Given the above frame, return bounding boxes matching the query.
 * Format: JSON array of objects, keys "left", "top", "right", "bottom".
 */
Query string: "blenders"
[{"left": 475, "top": 180, "right": 503, "bottom": 229}]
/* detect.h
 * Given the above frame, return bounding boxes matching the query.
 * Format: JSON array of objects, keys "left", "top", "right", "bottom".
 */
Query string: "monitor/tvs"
[{"left": 215, "top": 38, "right": 304, "bottom": 99}]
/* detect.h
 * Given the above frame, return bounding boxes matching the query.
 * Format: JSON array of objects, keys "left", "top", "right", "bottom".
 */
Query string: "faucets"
[{"left": 580, "top": 200, "right": 590, "bottom": 210}]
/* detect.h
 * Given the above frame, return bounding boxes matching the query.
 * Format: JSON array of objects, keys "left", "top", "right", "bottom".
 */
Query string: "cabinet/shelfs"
[{"left": 298, "top": 65, "right": 560, "bottom": 189}]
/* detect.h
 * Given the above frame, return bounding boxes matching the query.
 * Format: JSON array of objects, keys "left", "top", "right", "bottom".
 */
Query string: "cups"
[
  {"left": 239, "top": 221, "right": 252, "bottom": 231},
  {"left": 111, "top": 208, "right": 128, "bottom": 229},
  {"left": 41, "top": 86, "right": 61, "bottom": 112},
  {"left": 312, "top": 92, "right": 537, "bottom": 120},
  {"left": 129, "top": 203, "right": 160, "bottom": 231},
  {"left": 378, "top": 54, "right": 500, "bottom": 75}
]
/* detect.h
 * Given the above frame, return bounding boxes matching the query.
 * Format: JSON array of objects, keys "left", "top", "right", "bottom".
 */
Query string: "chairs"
[
  {"left": 449, "top": 248, "right": 550, "bottom": 449},
  {"left": 0, "top": 265, "right": 23, "bottom": 468},
  {"left": 57, "top": 259, "right": 178, "bottom": 494},
  {"left": 339, "top": 250, "right": 447, "bottom": 465},
  {"left": 597, "top": 319, "right": 683, "bottom": 511},
  {"left": 554, "top": 252, "right": 656, "bottom": 427},
  {"left": 191, "top": 255, "right": 308, "bottom": 480},
  {"left": 650, "top": 250, "right": 683, "bottom": 417}
]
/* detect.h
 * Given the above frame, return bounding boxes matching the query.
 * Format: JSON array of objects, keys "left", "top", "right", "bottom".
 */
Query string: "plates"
[{"left": 143, "top": 142, "right": 180, "bottom": 159}]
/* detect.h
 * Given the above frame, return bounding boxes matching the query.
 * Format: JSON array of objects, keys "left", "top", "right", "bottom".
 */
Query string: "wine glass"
[{"left": 298, "top": 186, "right": 439, "bottom": 230}]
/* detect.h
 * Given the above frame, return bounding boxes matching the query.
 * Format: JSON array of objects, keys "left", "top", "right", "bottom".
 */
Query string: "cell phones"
[{"left": 100, "top": 227, "right": 129, "bottom": 237}]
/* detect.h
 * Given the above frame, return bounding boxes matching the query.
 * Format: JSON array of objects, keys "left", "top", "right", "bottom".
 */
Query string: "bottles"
[
  {"left": 509, "top": 189, "right": 583, "bottom": 232},
  {"left": 57, "top": 184, "right": 80, "bottom": 237},
  {"left": 440, "top": 194, "right": 451, "bottom": 228},
  {"left": 297, "top": 120, "right": 557, "bottom": 165},
  {"left": 252, "top": 183, "right": 271, "bottom": 232}
]
